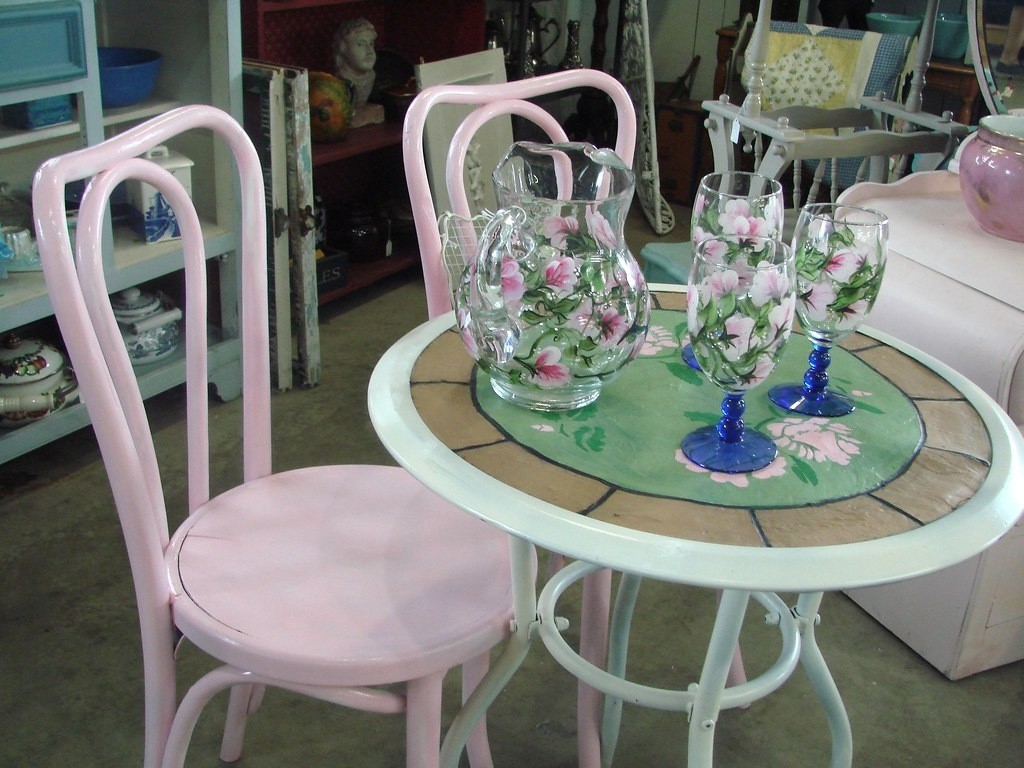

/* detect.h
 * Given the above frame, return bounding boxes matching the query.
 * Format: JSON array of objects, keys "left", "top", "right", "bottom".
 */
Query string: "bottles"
[
  {"left": 558, "top": 19, "right": 584, "bottom": 71},
  {"left": 958, "top": 113, "right": 1024, "bottom": 243}
]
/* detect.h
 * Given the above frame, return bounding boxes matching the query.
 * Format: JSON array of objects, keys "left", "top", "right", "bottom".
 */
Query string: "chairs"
[
  {"left": 403, "top": 67, "right": 751, "bottom": 768},
  {"left": 31, "top": 104, "right": 515, "bottom": 768}
]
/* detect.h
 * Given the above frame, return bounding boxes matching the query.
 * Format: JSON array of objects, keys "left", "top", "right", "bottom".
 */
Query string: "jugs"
[
  {"left": 0, "top": 332, "right": 80, "bottom": 429},
  {"left": 108, "top": 287, "right": 183, "bottom": 366},
  {"left": 453, "top": 139, "right": 652, "bottom": 414},
  {"left": 504, "top": 5, "right": 561, "bottom": 81}
]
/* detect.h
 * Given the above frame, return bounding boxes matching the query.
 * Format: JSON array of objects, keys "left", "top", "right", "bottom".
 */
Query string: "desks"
[
  {"left": 695, "top": 24, "right": 979, "bottom": 192},
  {"left": 367, "top": 283, "right": 1024, "bottom": 768}
]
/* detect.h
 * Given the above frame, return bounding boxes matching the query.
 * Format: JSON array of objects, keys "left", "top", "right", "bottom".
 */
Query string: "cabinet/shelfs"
[
  {"left": 241, "top": 0, "right": 487, "bottom": 323},
  {"left": 832, "top": 169, "right": 1024, "bottom": 683},
  {"left": 0, "top": 0, "right": 243, "bottom": 464}
]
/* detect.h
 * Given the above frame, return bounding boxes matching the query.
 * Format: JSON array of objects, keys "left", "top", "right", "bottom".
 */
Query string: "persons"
[
  {"left": 818, "top": 0, "right": 873, "bottom": 31},
  {"left": 995, "top": 0, "right": 1024, "bottom": 80},
  {"left": 332, "top": 18, "right": 376, "bottom": 86}
]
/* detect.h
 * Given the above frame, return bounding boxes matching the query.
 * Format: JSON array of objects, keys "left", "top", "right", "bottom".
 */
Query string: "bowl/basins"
[
  {"left": 920, "top": 11, "right": 969, "bottom": 59},
  {"left": 95, "top": 45, "right": 164, "bottom": 109},
  {"left": 865, "top": 12, "right": 922, "bottom": 35}
]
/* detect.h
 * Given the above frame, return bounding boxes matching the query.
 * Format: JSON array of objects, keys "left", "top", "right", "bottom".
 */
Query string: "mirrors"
[{"left": 967, "top": 0, "right": 1024, "bottom": 117}]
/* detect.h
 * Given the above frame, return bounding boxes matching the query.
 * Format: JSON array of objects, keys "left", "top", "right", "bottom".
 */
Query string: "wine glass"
[
  {"left": 681, "top": 171, "right": 784, "bottom": 374},
  {"left": 679, "top": 233, "right": 798, "bottom": 475},
  {"left": 768, "top": 201, "right": 888, "bottom": 417}
]
